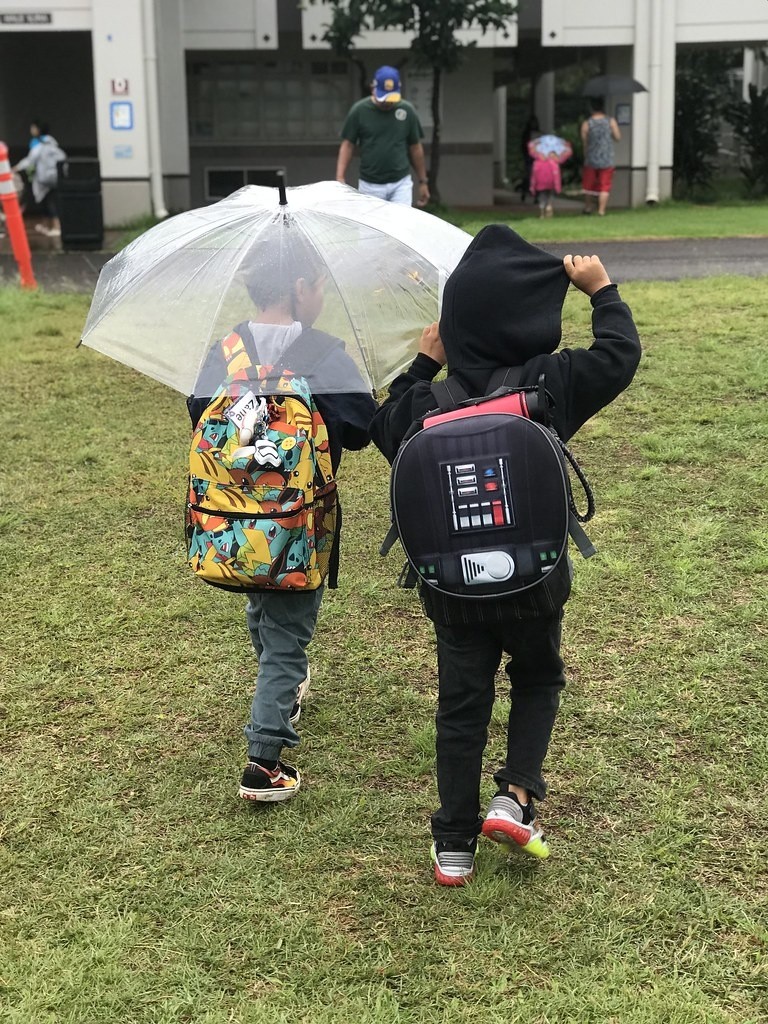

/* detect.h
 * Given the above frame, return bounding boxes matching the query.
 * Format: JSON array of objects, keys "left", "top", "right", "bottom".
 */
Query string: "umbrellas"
[
  {"left": 575, "top": 73, "right": 646, "bottom": 114},
  {"left": 528, "top": 159, "right": 562, "bottom": 219},
  {"left": 527, "top": 133, "right": 573, "bottom": 164},
  {"left": 75, "top": 171, "right": 474, "bottom": 405}
]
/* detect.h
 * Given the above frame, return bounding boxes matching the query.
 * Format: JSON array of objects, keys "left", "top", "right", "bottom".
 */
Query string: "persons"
[
  {"left": 336, "top": 65, "right": 431, "bottom": 208},
  {"left": 580, "top": 97, "right": 622, "bottom": 216},
  {"left": 13, "top": 118, "right": 67, "bottom": 254},
  {"left": 521, "top": 115, "right": 539, "bottom": 205},
  {"left": 185, "top": 229, "right": 379, "bottom": 802},
  {"left": 368, "top": 224, "right": 642, "bottom": 886}
]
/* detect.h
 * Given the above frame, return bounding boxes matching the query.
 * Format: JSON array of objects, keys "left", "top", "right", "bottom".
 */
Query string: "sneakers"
[
  {"left": 239, "top": 753, "right": 301, "bottom": 801},
  {"left": 481, "top": 796, "right": 550, "bottom": 860},
  {"left": 290, "top": 665, "right": 311, "bottom": 723},
  {"left": 430, "top": 835, "right": 480, "bottom": 886}
]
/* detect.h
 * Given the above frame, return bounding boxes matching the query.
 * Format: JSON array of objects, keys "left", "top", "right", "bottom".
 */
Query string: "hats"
[{"left": 374, "top": 66, "right": 403, "bottom": 103}]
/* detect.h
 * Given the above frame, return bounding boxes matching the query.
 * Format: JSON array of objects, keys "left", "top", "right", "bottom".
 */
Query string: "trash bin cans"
[{"left": 57, "top": 158, "right": 103, "bottom": 250}]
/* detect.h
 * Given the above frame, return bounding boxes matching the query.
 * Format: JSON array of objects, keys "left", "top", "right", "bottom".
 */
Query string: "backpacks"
[
  {"left": 183, "top": 326, "right": 339, "bottom": 591},
  {"left": 382, "top": 368, "right": 599, "bottom": 599}
]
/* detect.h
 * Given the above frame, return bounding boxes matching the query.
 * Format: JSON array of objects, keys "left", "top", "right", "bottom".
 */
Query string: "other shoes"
[
  {"left": 35, "top": 222, "right": 62, "bottom": 237},
  {"left": 546, "top": 205, "right": 555, "bottom": 217},
  {"left": 583, "top": 209, "right": 592, "bottom": 214}
]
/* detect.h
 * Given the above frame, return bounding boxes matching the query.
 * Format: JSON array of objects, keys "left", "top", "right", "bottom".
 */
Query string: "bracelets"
[{"left": 415, "top": 178, "right": 429, "bottom": 184}]
